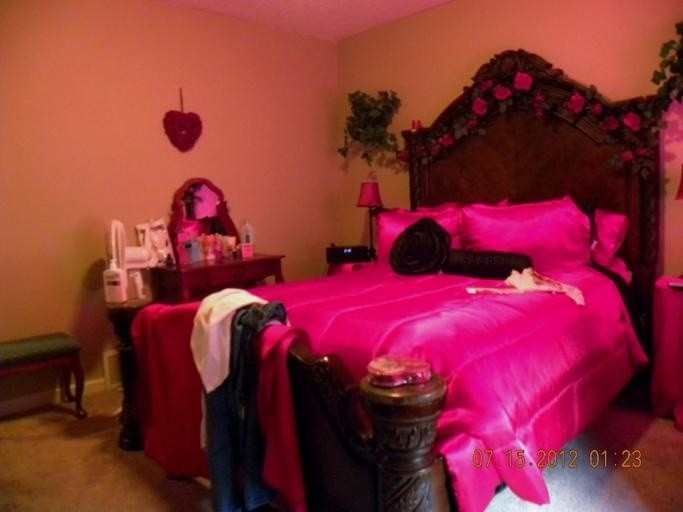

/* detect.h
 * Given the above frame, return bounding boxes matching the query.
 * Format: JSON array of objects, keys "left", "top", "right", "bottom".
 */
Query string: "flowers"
[{"left": 395, "top": 65, "right": 661, "bottom": 180}]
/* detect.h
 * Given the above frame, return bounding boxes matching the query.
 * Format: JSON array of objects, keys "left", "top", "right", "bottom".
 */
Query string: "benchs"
[{"left": 1, "top": 332, "right": 88, "bottom": 418}]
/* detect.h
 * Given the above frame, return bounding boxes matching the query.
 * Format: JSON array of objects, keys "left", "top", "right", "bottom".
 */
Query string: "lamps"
[{"left": 357, "top": 181, "right": 384, "bottom": 254}]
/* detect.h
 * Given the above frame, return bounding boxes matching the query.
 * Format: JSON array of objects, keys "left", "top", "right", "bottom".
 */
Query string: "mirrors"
[{"left": 169, "top": 179, "right": 241, "bottom": 260}]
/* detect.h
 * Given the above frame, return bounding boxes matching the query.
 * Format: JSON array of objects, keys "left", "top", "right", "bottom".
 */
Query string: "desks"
[{"left": 155, "top": 253, "right": 285, "bottom": 304}]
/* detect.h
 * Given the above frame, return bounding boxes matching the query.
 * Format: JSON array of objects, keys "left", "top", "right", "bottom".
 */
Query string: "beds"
[{"left": 109, "top": 48, "right": 663, "bottom": 511}]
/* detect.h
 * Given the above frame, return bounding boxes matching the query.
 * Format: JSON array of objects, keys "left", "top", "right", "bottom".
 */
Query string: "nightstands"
[
  {"left": 325, "top": 260, "right": 374, "bottom": 275},
  {"left": 655, "top": 274, "right": 683, "bottom": 430}
]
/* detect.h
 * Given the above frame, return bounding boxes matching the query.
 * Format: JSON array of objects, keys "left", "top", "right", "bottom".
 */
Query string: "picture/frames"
[{"left": 137, "top": 219, "right": 177, "bottom": 265}]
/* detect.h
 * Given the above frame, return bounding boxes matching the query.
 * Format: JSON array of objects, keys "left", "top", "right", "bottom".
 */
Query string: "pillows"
[
  {"left": 589, "top": 209, "right": 626, "bottom": 270},
  {"left": 461, "top": 196, "right": 591, "bottom": 275},
  {"left": 376, "top": 204, "right": 460, "bottom": 260}
]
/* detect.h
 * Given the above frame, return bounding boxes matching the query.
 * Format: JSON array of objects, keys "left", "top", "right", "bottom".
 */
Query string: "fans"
[{"left": 106, "top": 218, "right": 159, "bottom": 300}]
